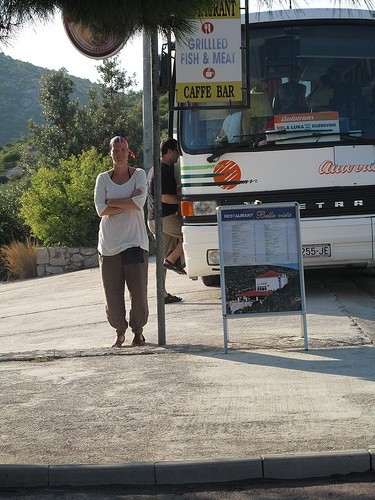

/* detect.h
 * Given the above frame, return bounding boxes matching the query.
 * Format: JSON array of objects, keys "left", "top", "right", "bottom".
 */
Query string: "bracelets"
[{"left": 105, "top": 200, "right": 108, "bottom": 204}]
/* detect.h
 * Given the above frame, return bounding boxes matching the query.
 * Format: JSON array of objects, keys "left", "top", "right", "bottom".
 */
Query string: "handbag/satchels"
[{"left": 122, "top": 246, "right": 145, "bottom": 263}]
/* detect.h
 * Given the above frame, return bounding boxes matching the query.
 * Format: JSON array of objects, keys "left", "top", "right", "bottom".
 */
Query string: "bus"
[{"left": 153, "top": 8, "right": 375, "bottom": 287}]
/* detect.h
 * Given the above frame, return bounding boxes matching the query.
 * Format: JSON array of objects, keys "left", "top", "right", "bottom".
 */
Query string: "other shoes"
[
  {"left": 131, "top": 334, "right": 145, "bottom": 347},
  {"left": 112, "top": 334, "right": 125, "bottom": 348}
]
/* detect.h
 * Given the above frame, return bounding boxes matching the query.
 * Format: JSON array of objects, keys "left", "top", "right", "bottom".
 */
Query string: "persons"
[
  {"left": 146, "top": 137, "right": 188, "bottom": 303},
  {"left": 217, "top": 65, "right": 375, "bottom": 142},
  {"left": 94, "top": 136, "right": 149, "bottom": 348}
]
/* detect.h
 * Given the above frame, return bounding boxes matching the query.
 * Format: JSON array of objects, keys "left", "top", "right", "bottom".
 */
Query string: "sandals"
[
  {"left": 164, "top": 293, "right": 182, "bottom": 304},
  {"left": 163, "top": 258, "right": 187, "bottom": 275}
]
